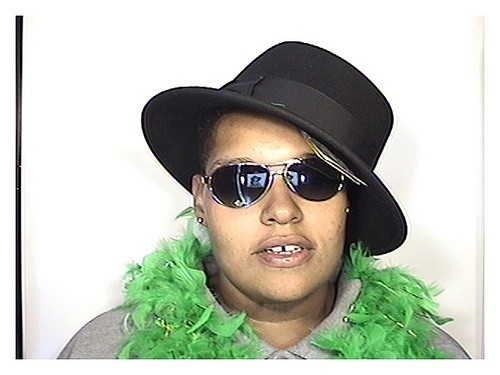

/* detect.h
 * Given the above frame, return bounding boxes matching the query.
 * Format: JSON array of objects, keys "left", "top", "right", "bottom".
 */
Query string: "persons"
[{"left": 51, "top": 40, "right": 476, "bottom": 359}]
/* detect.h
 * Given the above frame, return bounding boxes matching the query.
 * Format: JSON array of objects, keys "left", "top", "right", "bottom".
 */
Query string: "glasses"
[{"left": 202, "top": 156, "right": 349, "bottom": 209}]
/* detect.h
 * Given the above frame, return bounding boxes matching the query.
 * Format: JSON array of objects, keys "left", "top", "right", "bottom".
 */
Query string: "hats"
[{"left": 142, "top": 40, "right": 407, "bottom": 256}]
